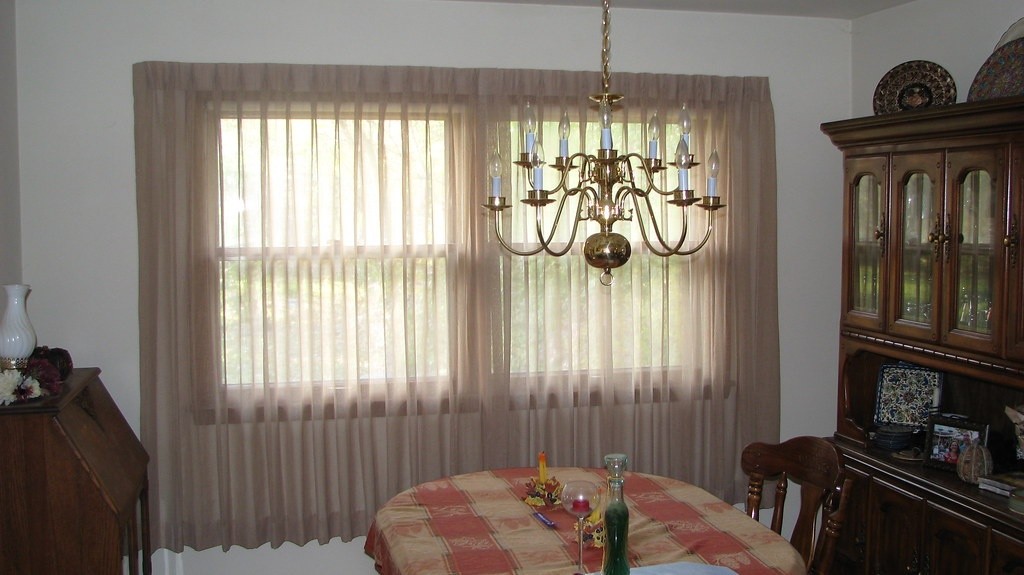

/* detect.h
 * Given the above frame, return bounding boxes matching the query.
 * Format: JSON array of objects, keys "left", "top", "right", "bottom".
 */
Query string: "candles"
[
  {"left": 571, "top": 496, "right": 589, "bottom": 511},
  {"left": 539, "top": 451, "right": 547, "bottom": 483}
]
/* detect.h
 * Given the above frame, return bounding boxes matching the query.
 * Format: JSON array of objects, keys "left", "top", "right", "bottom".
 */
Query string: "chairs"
[{"left": 741, "top": 436, "right": 854, "bottom": 575}]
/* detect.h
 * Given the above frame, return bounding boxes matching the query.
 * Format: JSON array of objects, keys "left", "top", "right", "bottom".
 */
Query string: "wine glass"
[{"left": 561, "top": 481, "right": 600, "bottom": 575}]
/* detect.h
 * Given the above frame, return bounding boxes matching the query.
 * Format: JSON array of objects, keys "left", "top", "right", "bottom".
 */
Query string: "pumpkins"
[{"left": 956, "top": 436, "right": 993, "bottom": 484}]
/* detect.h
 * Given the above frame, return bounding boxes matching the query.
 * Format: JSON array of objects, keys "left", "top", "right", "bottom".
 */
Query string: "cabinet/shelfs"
[
  {"left": 819, "top": 98, "right": 1024, "bottom": 575},
  {"left": 0, "top": 368, "right": 153, "bottom": 575}
]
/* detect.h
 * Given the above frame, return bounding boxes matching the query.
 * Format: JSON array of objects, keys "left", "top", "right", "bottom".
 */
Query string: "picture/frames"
[{"left": 923, "top": 414, "right": 988, "bottom": 475}]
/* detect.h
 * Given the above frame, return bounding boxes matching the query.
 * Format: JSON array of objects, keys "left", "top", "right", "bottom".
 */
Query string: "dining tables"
[{"left": 362, "top": 466, "right": 806, "bottom": 575}]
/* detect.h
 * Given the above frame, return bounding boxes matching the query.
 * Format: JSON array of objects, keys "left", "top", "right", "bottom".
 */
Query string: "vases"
[{"left": 0, "top": 283, "right": 37, "bottom": 369}]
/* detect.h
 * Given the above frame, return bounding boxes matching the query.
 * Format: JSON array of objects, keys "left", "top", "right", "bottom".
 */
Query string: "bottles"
[
  {"left": 601, "top": 454, "right": 632, "bottom": 575},
  {"left": 0, "top": 284, "right": 38, "bottom": 370}
]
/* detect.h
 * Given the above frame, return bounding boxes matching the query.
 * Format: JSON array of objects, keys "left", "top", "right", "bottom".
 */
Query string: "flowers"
[{"left": 0, "top": 356, "right": 62, "bottom": 406}]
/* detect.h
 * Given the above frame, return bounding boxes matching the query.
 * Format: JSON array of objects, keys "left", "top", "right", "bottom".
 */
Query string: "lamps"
[{"left": 480, "top": 0, "right": 728, "bottom": 286}]
